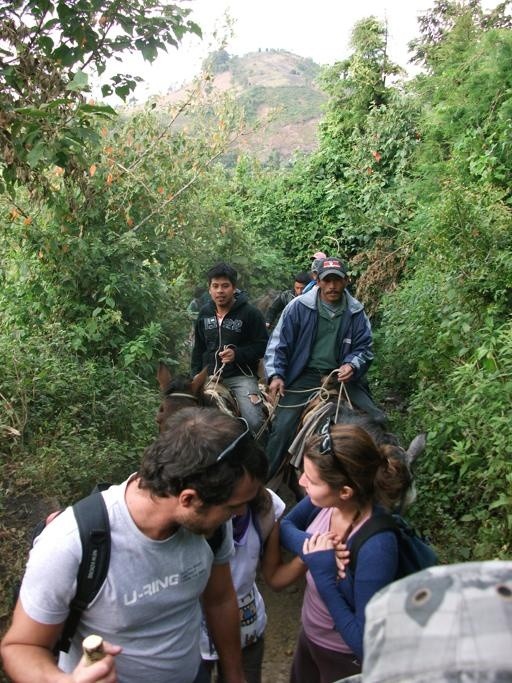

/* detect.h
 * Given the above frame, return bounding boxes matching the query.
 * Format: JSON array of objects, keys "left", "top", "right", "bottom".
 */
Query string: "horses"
[
  {"left": 284, "top": 389, "right": 428, "bottom": 519},
  {"left": 155, "top": 358, "right": 273, "bottom": 435}
]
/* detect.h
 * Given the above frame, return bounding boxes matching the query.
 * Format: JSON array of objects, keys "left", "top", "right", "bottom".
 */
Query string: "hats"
[
  {"left": 317, "top": 256, "right": 347, "bottom": 279},
  {"left": 332, "top": 558, "right": 512, "bottom": 683},
  {"left": 310, "top": 251, "right": 326, "bottom": 259},
  {"left": 311, "top": 258, "right": 325, "bottom": 271}
]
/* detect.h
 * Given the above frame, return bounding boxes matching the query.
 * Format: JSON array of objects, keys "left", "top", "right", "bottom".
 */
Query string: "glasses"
[
  {"left": 217, "top": 416, "right": 253, "bottom": 464},
  {"left": 318, "top": 418, "right": 356, "bottom": 486}
]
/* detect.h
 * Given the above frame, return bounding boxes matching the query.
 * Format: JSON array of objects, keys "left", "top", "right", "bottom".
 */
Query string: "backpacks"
[
  {"left": 32, "top": 481, "right": 227, "bottom": 651},
  {"left": 349, "top": 513, "right": 439, "bottom": 594}
]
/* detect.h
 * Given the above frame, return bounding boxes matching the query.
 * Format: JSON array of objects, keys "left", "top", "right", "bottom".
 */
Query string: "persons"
[
  {"left": 273, "top": 415, "right": 412, "bottom": 683},
  {"left": 262, "top": 270, "right": 313, "bottom": 330},
  {"left": 1, "top": 402, "right": 269, "bottom": 681},
  {"left": 195, "top": 486, "right": 351, "bottom": 683},
  {"left": 186, "top": 261, "right": 278, "bottom": 448},
  {"left": 257, "top": 257, "right": 391, "bottom": 476},
  {"left": 303, "top": 250, "right": 330, "bottom": 280}
]
